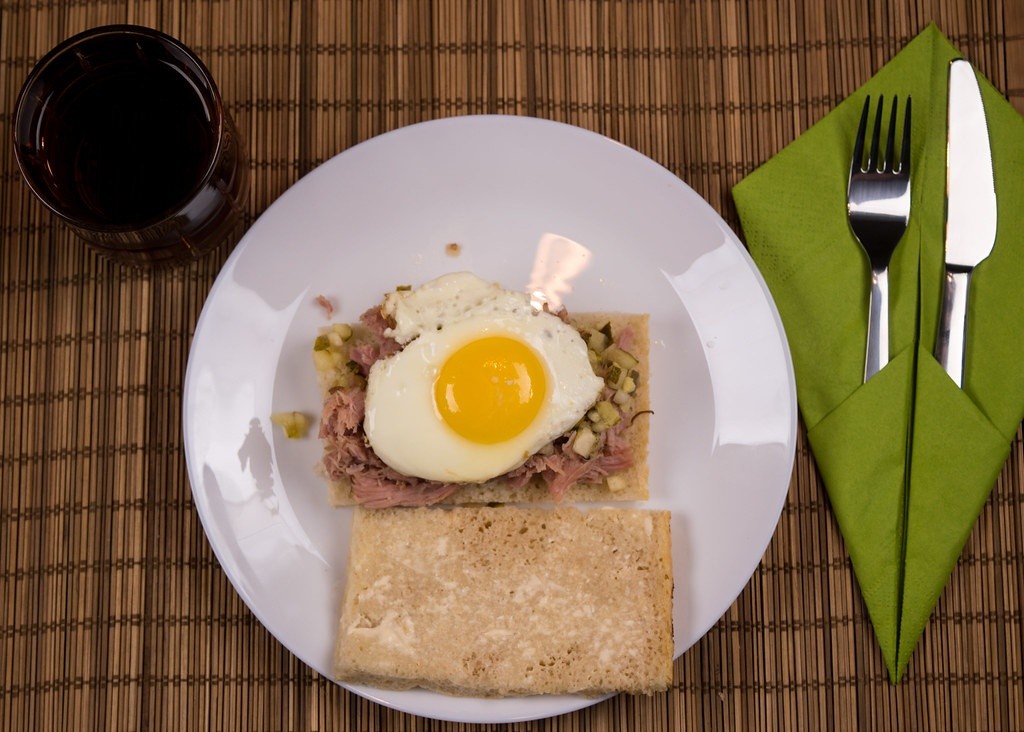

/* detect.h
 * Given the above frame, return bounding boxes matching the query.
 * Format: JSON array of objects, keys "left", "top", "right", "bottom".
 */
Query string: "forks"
[{"left": 845, "top": 92, "right": 915, "bottom": 386}]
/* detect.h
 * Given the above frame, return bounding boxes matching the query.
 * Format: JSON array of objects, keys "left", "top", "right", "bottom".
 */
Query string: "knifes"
[{"left": 927, "top": 56, "right": 999, "bottom": 391}]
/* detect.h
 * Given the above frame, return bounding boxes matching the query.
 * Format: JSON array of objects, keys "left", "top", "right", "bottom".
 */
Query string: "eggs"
[{"left": 364, "top": 270, "right": 605, "bottom": 484}]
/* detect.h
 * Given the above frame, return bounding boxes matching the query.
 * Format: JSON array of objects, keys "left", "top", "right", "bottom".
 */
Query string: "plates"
[{"left": 179, "top": 112, "right": 798, "bottom": 727}]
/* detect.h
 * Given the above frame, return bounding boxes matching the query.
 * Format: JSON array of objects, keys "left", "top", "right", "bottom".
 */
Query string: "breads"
[
  {"left": 329, "top": 312, "right": 652, "bottom": 501},
  {"left": 335, "top": 505, "right": 674, "bottom": 694}
]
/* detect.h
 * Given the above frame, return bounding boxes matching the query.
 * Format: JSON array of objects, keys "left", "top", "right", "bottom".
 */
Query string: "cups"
[{"left": 10, "top": 24, "right": 250, "bottom": 272}]
